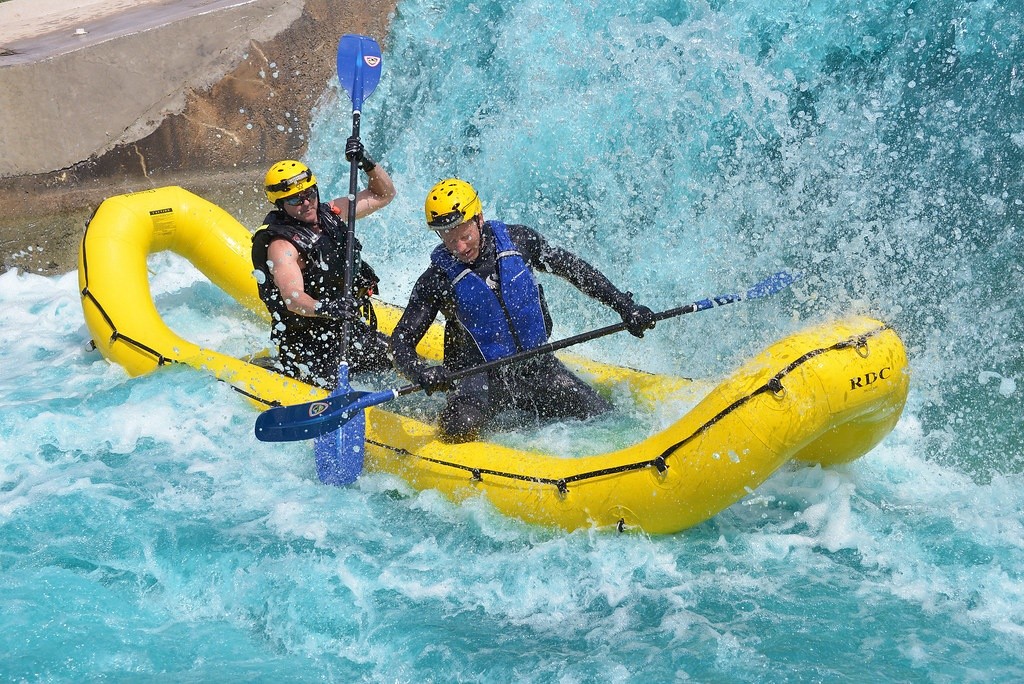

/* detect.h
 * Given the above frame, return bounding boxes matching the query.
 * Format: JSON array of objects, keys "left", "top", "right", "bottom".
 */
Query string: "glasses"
[{"left": 286, "top": 190, "right": 318, "bottom": 206}]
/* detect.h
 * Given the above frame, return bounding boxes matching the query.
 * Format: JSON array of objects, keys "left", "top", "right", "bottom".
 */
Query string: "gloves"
[
  {"left": 418, "top": 365, "right": 457, "bottom": 395},
  {"left": 315, "top": 298, "right": 358, "bottom": 321},
  {"left": 621, "top": 302, "right": 655, "bottom": 338},
  {"left": 345, "top": 136, "right": 369, "bottom": 169}
]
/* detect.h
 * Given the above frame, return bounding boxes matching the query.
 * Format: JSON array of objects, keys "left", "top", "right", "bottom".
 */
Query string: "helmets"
[
  {"left": 264, "top": 160, "right": 316, "bottom": 205},
  {"left": 425, "top": 179, "right": 482, "bottom": 231}
]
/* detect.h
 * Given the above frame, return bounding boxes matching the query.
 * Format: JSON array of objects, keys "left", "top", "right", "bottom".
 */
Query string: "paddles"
[
  {"left": 253, "top": 267, "right": 806, "bottom": 446},
  {"left": 311, "top": 32, "right": 386, "bottom": 489}
]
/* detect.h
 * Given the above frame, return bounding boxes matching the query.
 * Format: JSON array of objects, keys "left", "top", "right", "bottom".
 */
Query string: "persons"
[
  {"left": 250, "top": 136, "right": 397, "bottom": 388},
  {"left": 390, "top": 176, "right": 656, "bottom": 441}
]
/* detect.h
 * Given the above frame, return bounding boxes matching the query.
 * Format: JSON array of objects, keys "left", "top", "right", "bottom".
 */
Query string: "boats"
[{"left": 77, "top": 185, "right": 911, "bottom": 536}]
